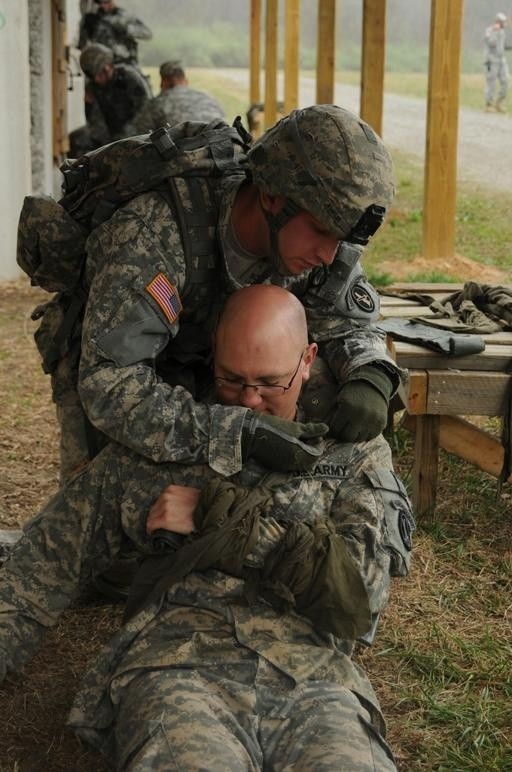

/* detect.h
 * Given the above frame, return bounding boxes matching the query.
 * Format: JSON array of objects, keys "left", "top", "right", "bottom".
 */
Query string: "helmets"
[
  {"left": 247, "top": 104, "right": 395, "bottom": 244},
  {"left": 80, "top": 43, "right": 113, "bottom": 77}
]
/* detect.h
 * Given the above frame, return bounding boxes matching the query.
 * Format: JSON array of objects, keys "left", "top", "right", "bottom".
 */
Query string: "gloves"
[
  {"left": 324, "top": 365, "right": 393, "bottom": 444},
  {"left": 241, "top": 410, "right": 329, "bottom": 471}
]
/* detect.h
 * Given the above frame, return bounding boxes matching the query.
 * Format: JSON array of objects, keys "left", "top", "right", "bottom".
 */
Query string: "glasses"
[{"left": 211, "top": 349, "right": 303, "bottom": 396}]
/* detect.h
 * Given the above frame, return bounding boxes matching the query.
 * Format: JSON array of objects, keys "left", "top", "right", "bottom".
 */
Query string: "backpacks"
[{"left": 17, "top": 116, "right": 252, "bottom": 342}]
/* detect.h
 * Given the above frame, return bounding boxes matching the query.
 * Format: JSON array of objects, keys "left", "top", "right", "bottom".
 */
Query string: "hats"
[{"left": 159, "top": 60, "right": 182, "bottom": 87}]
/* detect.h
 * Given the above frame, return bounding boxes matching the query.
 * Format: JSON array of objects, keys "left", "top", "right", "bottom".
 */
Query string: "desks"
[{"left": 371, "top": 281, "right": 512, "bottom": 528}]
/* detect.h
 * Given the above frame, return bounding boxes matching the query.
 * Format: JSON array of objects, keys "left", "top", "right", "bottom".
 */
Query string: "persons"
[
  {"left": 482, "top": 11, "right": 512, "bottom": 115},
  {"left": 68, "top": 44, "right": 153, "bottom": 157},
  {"left": 77, "top": 1, "right": 153, "bottom": 99},
  {"left": 30, "top": 102, "right": 411, "bottom": 613},
  {"left": 114, "top": 58, "right": 240, "bottom": 140},
  {"left": 0, "top": 283, "right": 418, "bottom": 771}
]
